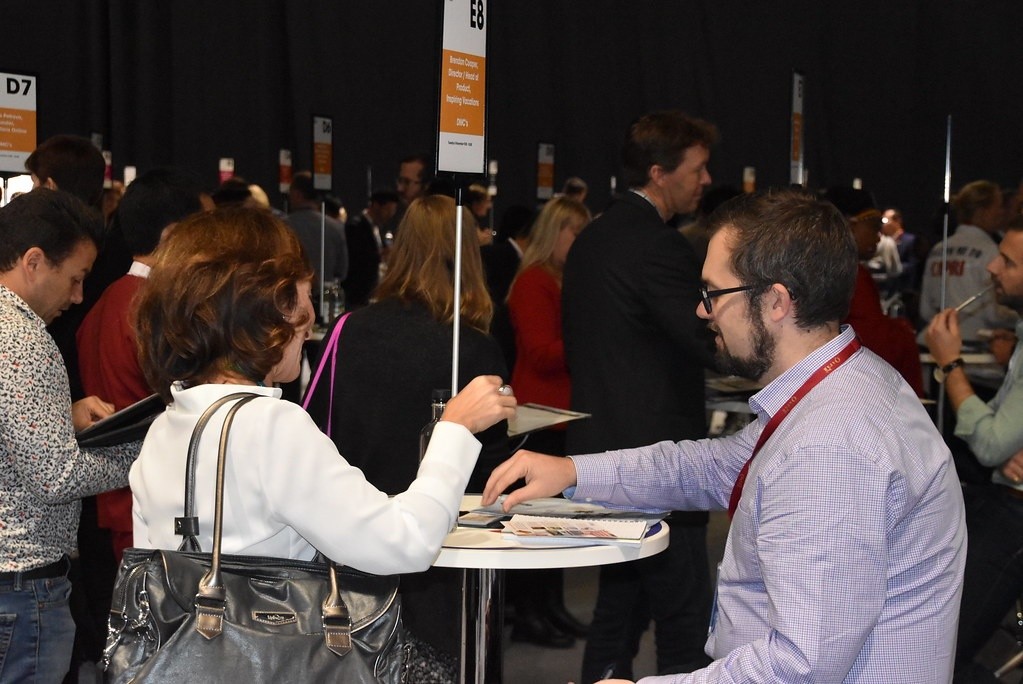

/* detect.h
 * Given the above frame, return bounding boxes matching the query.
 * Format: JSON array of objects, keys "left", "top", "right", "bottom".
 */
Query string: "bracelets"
[{"left": 934, "top": 356, "right": 964, "bottom": 382}]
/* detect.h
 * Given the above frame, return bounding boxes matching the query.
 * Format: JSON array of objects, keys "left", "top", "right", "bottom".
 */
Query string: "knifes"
[{"left": 955, "top": 283, "right": 995, "bottom": 312}]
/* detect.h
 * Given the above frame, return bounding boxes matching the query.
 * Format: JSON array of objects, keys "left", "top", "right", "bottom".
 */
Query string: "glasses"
[
  {"left": 397, "top": 176, "right": 419, "bottom": 188},
  {"left": 701, "top": 283, "right": 797, "bottom": 314}
]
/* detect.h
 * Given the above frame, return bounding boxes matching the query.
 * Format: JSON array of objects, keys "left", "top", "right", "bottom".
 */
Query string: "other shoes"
[{"left": 510, "top": 605, "right": 588, "bottom": 648}]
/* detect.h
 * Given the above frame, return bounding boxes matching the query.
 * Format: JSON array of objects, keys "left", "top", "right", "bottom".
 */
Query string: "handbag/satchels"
[{"left": 98, "top": 393, "right": 412, "bottom": 684}]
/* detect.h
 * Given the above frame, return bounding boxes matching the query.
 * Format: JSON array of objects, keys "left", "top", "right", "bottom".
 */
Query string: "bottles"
[
  {"left": 323, "top": 281, "right": 333, "bottom": 327},
  {"left": 419, "top": 389, "right": 451, "bottom": 465},
  {"left": 385, "top": 231, "right": 393, "bottom": 245},
  {"left": 329, "top": 273, "right": 344, "bottom": 325}
]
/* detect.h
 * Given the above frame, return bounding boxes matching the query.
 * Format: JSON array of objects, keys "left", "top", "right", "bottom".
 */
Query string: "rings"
[{"left": 499, "top": 386, "right": 512, "bottom": 395}]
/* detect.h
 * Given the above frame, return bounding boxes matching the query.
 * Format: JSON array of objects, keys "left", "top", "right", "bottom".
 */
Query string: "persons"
[
  {"left": 554, "top": 107, "right": 722, "bottom": 684},
  {"left": 0, "top": 134, "right": 617, "bottom": 684},
  {"left": 128, "top": 206, "right": 518, "bottom": 576},
  {"left": 823, "top": 176, "right": 1023, "bottom": 684},
  {"left": 0, "top": 187, "right": 145, "bottom": 684},
  {"left": 482, "top": 181, "right": 968, "bottom": 684}
]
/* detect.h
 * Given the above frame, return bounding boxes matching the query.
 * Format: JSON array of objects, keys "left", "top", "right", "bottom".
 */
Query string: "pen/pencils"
[{"left": 955, "top": 283, "right": 995, "bottom": 311}]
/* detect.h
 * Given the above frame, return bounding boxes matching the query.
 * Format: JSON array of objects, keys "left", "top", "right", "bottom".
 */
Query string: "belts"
[{"left": 0, "top": 561, "right": 69, "bottom": 582}]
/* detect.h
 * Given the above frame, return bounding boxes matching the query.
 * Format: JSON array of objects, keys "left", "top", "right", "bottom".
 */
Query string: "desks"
[{"left": 432, "top": 493, "right": 670, "bottom": 684}]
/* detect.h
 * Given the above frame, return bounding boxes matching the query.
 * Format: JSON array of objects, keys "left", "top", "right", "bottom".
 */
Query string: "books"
[{"left": 500, "top": 510, "right": 671, "bottom": 548}]
